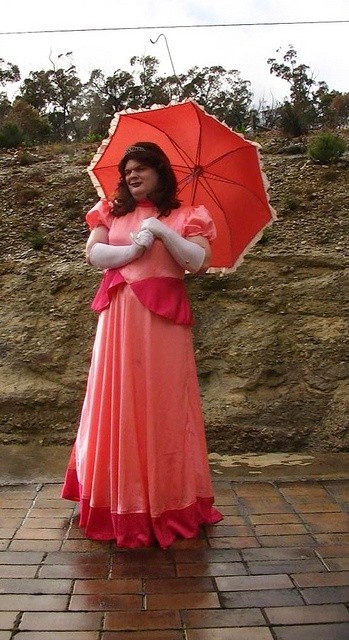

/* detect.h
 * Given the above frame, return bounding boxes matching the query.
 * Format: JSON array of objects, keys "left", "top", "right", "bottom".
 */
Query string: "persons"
[{"left": 61, "top": 141, "right": 223, "bottom": 548}]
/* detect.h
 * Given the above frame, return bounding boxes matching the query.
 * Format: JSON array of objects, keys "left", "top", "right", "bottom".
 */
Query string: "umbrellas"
[{"left": 87, "top": 98, "right": 278, "bottom": 272}]
